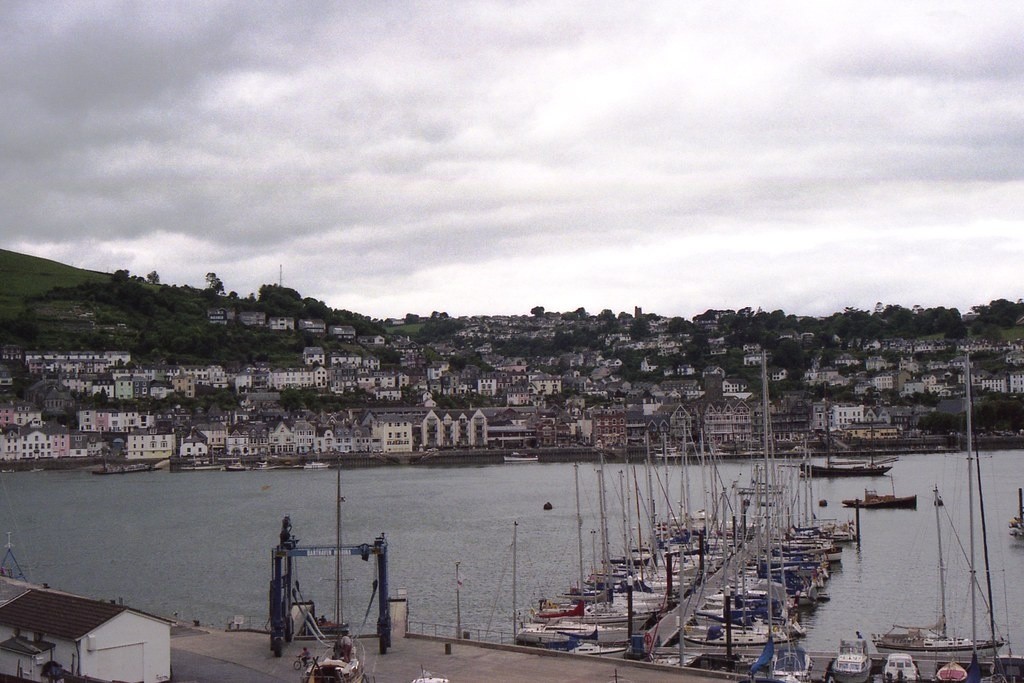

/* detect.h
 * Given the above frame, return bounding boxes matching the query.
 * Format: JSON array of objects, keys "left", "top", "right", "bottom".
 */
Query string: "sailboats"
[
  {"left": 797, "top": 379, "right": 894, "bottom": 478},
  {"left": 869, "top": 482, "right": 1006, "bottom": 657},
  {"left": 502, "top": 348, "right": 860, "bottom": 683},
  {"left": 293, "top": 453, "right": 351, "bottom": 635}
]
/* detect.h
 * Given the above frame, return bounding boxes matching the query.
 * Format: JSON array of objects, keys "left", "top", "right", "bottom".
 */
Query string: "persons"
[
  {"left": 340, "top": 632, "right": 352, "bottom": 663},
  {"left": 856, "top": 631, "right": 862, "bottom": 639},
  {"left": 300, "top": 647, "right": 312, "bottom": 667},
  {"left": 319, "top": 615, "right": 327, "bottom": 623}
]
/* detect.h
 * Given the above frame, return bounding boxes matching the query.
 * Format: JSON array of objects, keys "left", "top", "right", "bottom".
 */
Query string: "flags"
[{"left": 457, "top": 570, "right": 467, "bottom": 588}]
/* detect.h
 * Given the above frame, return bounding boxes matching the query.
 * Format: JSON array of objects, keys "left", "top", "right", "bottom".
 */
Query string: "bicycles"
[{"left": 293, "top": 654, "right": 313, "bottom": 671}]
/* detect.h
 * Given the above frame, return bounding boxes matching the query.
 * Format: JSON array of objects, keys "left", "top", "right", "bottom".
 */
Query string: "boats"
[
  {"left": 299, "top": 636, "right": 369, "bottom": 683},
  {"left": 841, "top": 489, "right": 918, "bottom": 510},
  {"left": 225, "top": 462, "right": 246, "bottom": 470},
  {"left": 882, "top": 651, "right": 918, "bottom": 682},
  {"left": 831, "top": 637, "right": 873, "bottom": 683},
  {"left": 302, "top": 460, "right": 329, "bottom": 470},
  {"left": 252, "top": 460, "right": 277, "bottom": 470},
  {"left": 503, "top": 451, "right": 539, "bottom": 461},
  {"left": 936, "top": 654, "right": 969, "bottom": 682}
]
